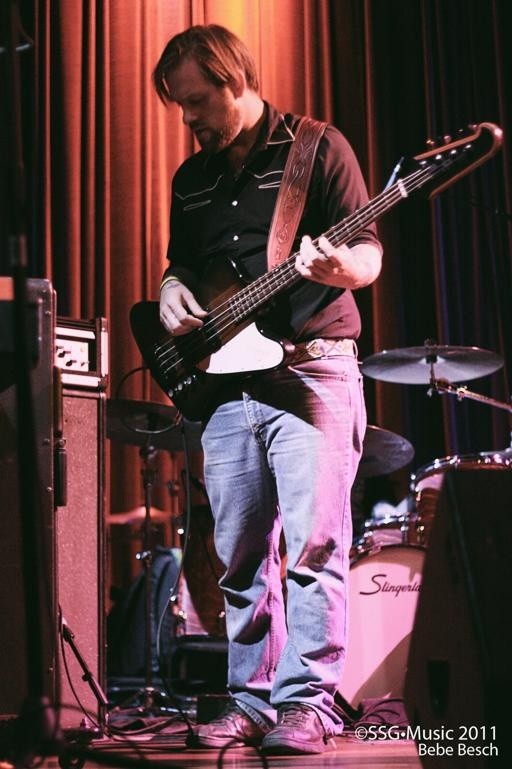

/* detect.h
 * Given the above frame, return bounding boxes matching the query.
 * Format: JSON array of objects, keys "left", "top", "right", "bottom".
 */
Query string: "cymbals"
[
  {"left": 104, "top": 399, "right": 204, "bottom": 450},
  {"left": 359, "top": 346, "right": 504, "bottom": 385},
  {"left": 357, "top": 425, "right": 413, "bottom": 473}
]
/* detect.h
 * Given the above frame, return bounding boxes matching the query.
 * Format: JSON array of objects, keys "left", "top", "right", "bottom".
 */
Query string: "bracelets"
[{"left": 160, "top": 275, "right": 178, "bottom": 291}]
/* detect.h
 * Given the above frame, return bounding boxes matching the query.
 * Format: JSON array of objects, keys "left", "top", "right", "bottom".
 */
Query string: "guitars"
[{"left": 130, "top": 121, "right": 504, "bottom": 424}]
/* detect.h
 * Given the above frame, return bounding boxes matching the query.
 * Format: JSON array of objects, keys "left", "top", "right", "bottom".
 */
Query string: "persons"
[{"left": 152, "top": 23, "right": 387, "bottom": 753}]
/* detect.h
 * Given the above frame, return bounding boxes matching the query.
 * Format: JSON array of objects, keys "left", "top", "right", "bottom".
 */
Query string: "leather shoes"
[
  {"left": 260, "top": 703, "right": 338, "bottom": 756},
  {"left": 186, "top": 706, "right": 265, "bottom": 749}
]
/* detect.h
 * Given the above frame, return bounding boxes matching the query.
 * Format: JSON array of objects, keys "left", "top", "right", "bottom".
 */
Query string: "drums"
[
  {"left": 403, "top": 450, "right": 510, "bottom": 546},
  {"left": 125, "top": 548, "right": 209, "bottom": 688},
  {"left": 278, "top": 514, "right": 432, "bottom": 727}
]
